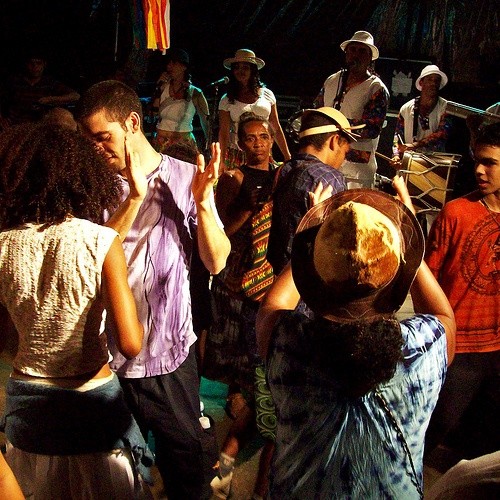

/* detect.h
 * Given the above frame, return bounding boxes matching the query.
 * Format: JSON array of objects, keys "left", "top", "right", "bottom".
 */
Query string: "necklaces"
[
  {"left": 169, "top": 82, "right": 185, "bottom": 99},
  {"left": 480, "top": 193, "right": 499, "bottom": 228}
]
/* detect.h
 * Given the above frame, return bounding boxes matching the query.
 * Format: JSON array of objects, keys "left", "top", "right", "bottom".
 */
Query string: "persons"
[
  {"left": 255, "top": 189, "right": 458, "bottom": 500},
  {"left": 75, "top": 79, "right": 231, "bottom": 500},
  {"left": 264, "top": 106, "right": 367, "bottom": 280},
  {"left": 27, "top": 102, "right": 77, "bottom": 128},
  {"left": 8, "top": 53, "right": 80, "bottom": 109},
  {"left": 312, "top": 31, "right": 390, "bottom": 190},
  {"left": 0, "top": 116, "right": 145, "bottom": 500},
  {"left": 200, "top": 111, "right": 280, "bottom": 499},
  {"left": 392, "top": 64, "right": 457, "bottom": 172},
  {"left": 425, "top": 121, "right": 500, "bottom": 468},
  {"left": 150, "top": 47, "right": 211, "bottom": 165},
  {"left": 216, "top": 49, "right": 294, "bottom": 169}
]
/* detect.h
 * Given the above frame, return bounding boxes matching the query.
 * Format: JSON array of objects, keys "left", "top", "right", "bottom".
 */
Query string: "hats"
[
  {"left": 223, "top": 49, "right": 265, "bottom": 70},
  {"left": 292, "top": 188, "right": 425, "bottom": 321},
  {"left": 162, "top": 48, "right": 188, "bottom": 67},
  {"left": 288, "top": 107, "right": 352, "bottom": 144},
  {"left": 340, "top": 31, "right": 379, "bottom": 61},
  {"left": 415, "top": 65, "right": 448, "bottom": 91}
]
